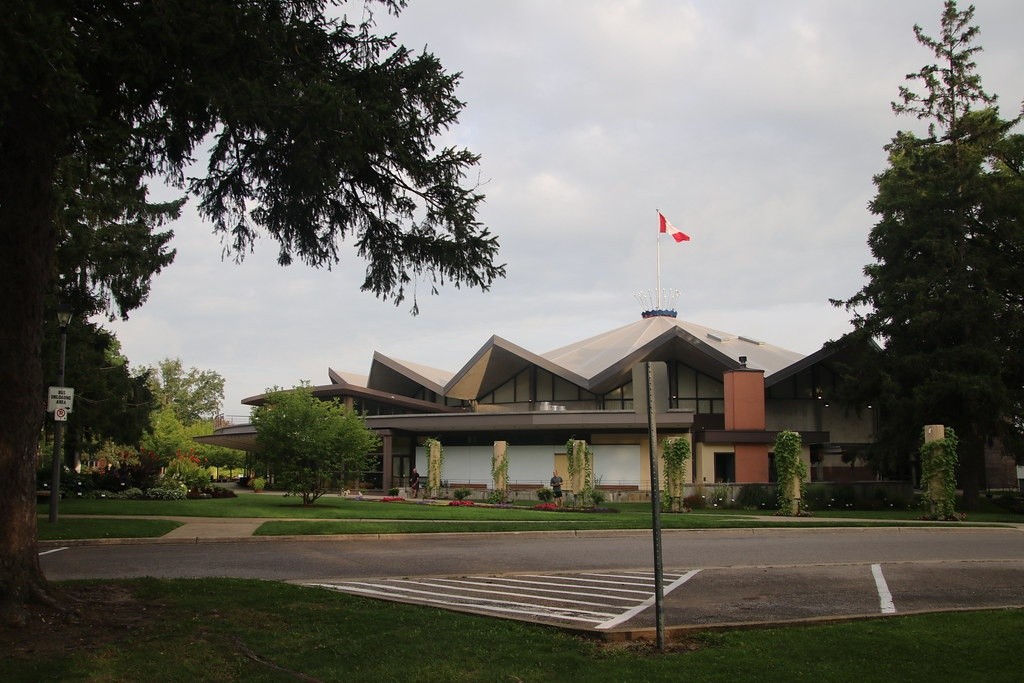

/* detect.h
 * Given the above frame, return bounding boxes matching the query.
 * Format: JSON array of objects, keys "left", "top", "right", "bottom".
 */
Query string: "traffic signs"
[{"left": 47, "top": 386, "right": 75, "bottom": 414}]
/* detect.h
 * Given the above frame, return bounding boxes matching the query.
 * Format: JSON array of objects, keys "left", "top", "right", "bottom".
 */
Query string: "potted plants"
[{"left": 251, "top": 475, "right": 267, "bottom": 493}]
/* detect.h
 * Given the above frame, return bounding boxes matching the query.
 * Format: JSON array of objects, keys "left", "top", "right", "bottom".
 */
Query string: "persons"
[
  {"left": 410, "top": 468, "right": 420, "bottom": 498},
  {"left": 550, "top": 470, "right": 564, "bottom": 509}
]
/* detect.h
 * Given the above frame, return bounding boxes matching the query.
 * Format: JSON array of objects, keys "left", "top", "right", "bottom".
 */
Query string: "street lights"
[{"left": 49, "top": 303, "right": 78, "bottom": 522}]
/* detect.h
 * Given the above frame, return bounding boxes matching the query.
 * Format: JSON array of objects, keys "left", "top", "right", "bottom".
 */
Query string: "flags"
[{"left": 658, "top": 212, "right": 691, "bottom": 243}]
